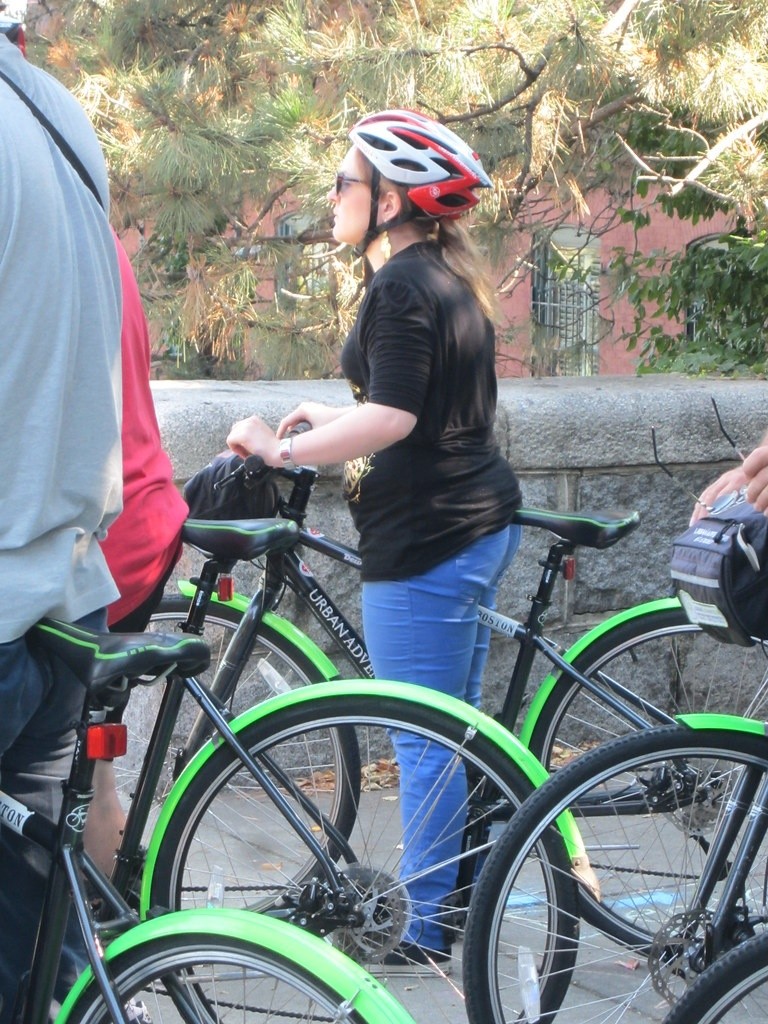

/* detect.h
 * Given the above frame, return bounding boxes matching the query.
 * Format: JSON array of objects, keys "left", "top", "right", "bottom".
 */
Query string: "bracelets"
[{"left": 280, "top": 438, "right": 296, "bottom": 470}]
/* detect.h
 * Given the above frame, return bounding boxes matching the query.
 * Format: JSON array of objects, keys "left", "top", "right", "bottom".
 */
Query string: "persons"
[
  {"left": 0, "top": 0, "right": 193, "bottom": 1024},
  {"left": 689, "top": 433, "right": 768, "bottom": 529},
  {"left": 228, "top": 110, "right": 522, "bottom": 980}
]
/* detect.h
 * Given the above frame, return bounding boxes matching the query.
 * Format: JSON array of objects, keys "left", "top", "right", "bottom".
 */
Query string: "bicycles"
[
  {"left": 0, "top": 519, "right": 768, "bottom": 1024},
  {"left": 154, "top": 419, "right": 768, "bottom": 973}
]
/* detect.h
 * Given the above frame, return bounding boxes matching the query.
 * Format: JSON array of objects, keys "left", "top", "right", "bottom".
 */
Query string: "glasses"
[
  {"left": 651, "top": 397, "right": 751, "bottom": 515},
  {"left": 335, "top": 171, "right": 371, "bottom": 197}
]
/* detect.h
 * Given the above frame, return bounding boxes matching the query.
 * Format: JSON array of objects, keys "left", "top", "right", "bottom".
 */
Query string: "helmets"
[{"left": 349, "top": 108, "right": 494, "bottom": 221}]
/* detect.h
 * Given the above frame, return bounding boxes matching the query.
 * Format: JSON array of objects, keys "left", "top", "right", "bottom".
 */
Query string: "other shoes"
[
  {"left": 49, "top": 998, "right": 153, "bottom": 1024},
  {"left": 332, "top": 932, "right": 452, "bottom": 977}
]
